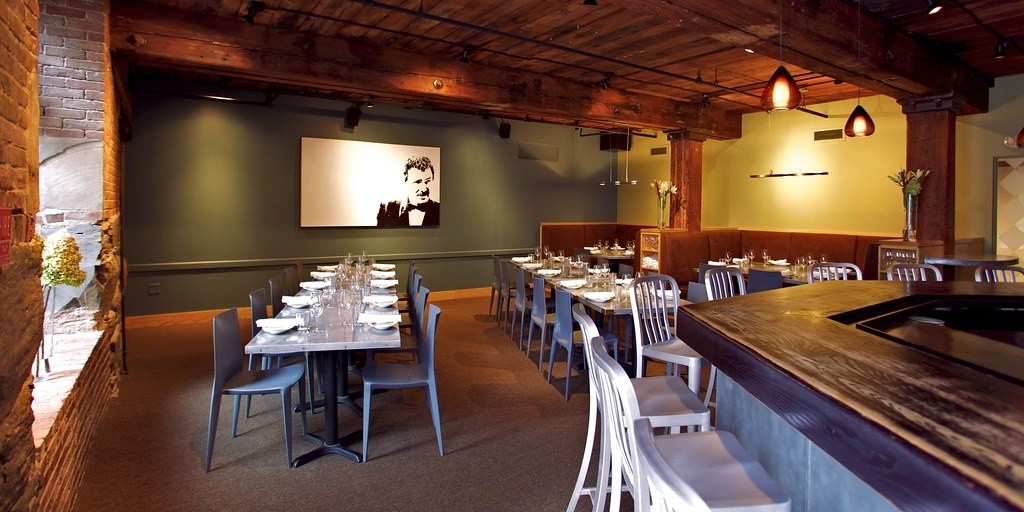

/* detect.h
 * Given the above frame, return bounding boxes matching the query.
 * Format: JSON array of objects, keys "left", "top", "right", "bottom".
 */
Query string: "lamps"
[
  {"left": 760, "top": 0, "right": 801, "bottom": 112},
  {"left": 844, "top": 0, "right": 876, "bottom": 137},
  {"left": 701, "top": 94, "right": 712, "bottom": 107},
  {"left": 994, "top": 39, "right": 1010, "bottom": 59},
  {"left": 458, "top": 45, "right": 473, "bottom": 62},
  {"left": 242, "top": 1, "right": 265, "bottom": 25},
  {"left": 600, "top": 126, "right": 638, "bottom": 187},
  {"left": 601, "top": 72, "right": 613, "bottom": 89},
  {"left": 1002, "top": 128, "right": 1024, "bottom": 148}
]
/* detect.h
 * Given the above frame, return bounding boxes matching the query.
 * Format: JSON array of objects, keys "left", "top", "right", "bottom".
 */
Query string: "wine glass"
[
  {"left": 881, "top": 250, "right": 917, "bottom": 273},
  {"left": 642, "top": 234, "right": 659, "bottom": 251},
  {"left": 724, "top": 249, "right": 828, "bottom": 278},
  {"left": 534, "top": 245, "right": 645, "bottom": 303},
  {"left": 309, "top": 250, "right": 369, "bottom": 333}
]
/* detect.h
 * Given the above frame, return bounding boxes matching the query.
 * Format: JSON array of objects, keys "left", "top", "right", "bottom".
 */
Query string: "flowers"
[
  {"left": 650, "top": 180, "right": 678, "bottom": 201},
  {"left": 34, "top": 229, "right": 87, "bottom": 288},
  {"left": 886, "top": 169, "right": 932, "bottom": 209}
]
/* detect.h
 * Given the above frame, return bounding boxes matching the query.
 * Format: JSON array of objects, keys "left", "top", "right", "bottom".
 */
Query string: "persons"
[{"left": 377, "top": 154, "right": 440, "bottom": 228}]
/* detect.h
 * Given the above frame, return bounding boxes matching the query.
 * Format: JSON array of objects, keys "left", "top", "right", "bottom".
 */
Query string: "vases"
[
  {"left": 659, "top": 198, "right": 665, "bottom": 230},
  {"left": 908, "top": 193, "right": 918, "bottom": 241}
]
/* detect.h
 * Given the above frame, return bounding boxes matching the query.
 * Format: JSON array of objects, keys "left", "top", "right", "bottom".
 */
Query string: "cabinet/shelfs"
[
  {"left": 640, "top": 228, "right": 687, "bottom": 277},
  {"left": 878, "top": 240, "right": 943, "bottom": 281}
]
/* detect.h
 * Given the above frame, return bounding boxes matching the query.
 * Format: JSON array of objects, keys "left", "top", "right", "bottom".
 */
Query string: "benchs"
[{"left": 539, "top": 223, "right": 900, "bottom": 301}]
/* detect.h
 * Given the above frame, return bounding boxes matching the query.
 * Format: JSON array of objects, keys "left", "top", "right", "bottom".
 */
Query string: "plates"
[
  {"left": 623, "top": 252, "right": 633, "bottom": 255},
  {"left": 583, "top": 247, "right": 598, "bottom": 250},
  {"left": 260, "top": 265, "right": 339, "bottom": 333},
  {"left": 368, "top": 264, "right": 398, "bottom": 329},
  {"left": 590, "top": 250, "right": 602, "bottom": 255},
  {"left": 511, "top": 256, "right": 681, "bottom": 303},
  {"left": 708, "top": 258, "right": 852, "bottom": 280},
  {"left": 610, "top": 248, "right": 625, "bottom": 250}
]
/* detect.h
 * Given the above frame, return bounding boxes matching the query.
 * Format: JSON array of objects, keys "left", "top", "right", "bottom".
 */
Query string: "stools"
[
  {"left": 807, "top": 262, "right": 1024, "bottom": 284},
  {"left": 566, "top": 268, "right": 791, "bottom": 512}
]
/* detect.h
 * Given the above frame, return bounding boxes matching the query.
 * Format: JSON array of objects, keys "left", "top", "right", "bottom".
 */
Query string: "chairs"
[{"left": 204, "top": 254, "right": 783, "bottom": 471}]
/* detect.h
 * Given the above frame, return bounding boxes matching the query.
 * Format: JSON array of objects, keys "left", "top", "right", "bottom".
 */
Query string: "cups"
[
  {"left": 641, "top": 253, "right": 658, "bottom": 269},
  {"left": 528, "top": 252, "right": 653, "bottom": 302},
  {"left": 719, "top": 253, "right": 818, "bottom": 279},
  {"left": 296, "top": 257, "right": 377, "bottom": 331},
  {"left": 594, "top": 239, "right": 636, "bottom": 255}
]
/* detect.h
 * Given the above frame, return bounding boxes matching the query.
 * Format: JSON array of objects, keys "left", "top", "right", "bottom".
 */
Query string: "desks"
[
  {"left": 690, "top": 259, "right": 869, "bottom": 285},
  {"left": 589, "top": 249, "right": 634, "bottom": 259},
  {"left": 924, "top": 253, "right": 1020, "bottom": 267},
  {"left": 507, "top": 259, "right": 695, "bottom": 378},
  {"left": 245, "top": 263, "right": 401, "bottom": 468}
]
